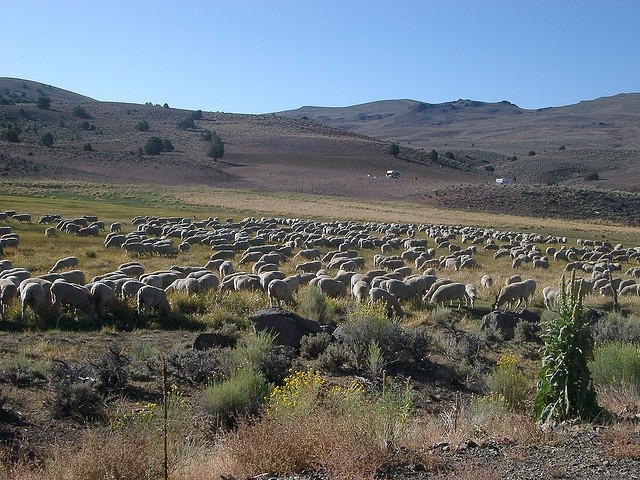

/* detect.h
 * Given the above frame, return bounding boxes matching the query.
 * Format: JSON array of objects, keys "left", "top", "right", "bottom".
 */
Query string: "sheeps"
[{"left": 0, "top": 210, "right": 640, "bottom": 320}]
